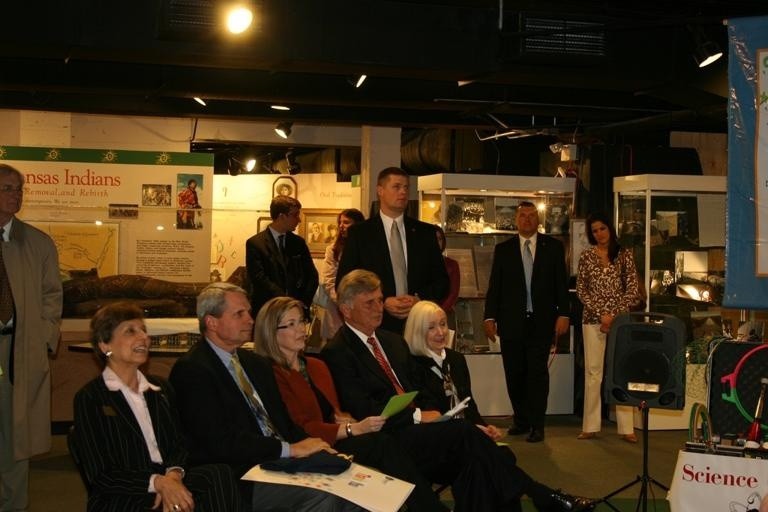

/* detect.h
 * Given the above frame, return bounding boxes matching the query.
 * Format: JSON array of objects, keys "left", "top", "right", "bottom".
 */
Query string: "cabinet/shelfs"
[
  {"left": 418, "top": 173, "right": 577, "bottom": 417},
  {"left": 612, "top": 174, "right": 740, "bottom": 430}
]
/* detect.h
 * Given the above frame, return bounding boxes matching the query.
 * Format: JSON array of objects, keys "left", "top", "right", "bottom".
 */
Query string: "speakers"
[{"left": 602, "top": 312, "right": 686, "bottom": 409}]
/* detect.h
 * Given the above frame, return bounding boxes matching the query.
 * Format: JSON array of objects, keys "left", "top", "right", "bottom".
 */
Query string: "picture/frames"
[
  {"left": 271, "top": 175, "right": 298, "bottom": 200},
  {"left": 256, "top": 216, "right": 274, "bottom": 235},
  {"left": 297, "top": 208, "right": 345, "bottom": 259}
]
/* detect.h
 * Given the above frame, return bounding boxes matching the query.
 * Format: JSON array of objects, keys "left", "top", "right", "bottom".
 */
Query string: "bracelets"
[{"left": 346, "top": 422, "right": 353, "bottom": 439}]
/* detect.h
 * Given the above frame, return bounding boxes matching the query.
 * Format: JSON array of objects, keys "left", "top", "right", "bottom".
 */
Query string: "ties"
[
  {"left": 231, "top": 356, "right": 283, "bottom": 440},
  {"left": 523, "top": 240, "right": 534, "bottom": 313},
  {"left": 0, "top": 228, "right": 13, "bottom": 325},
  {"left": 368, "top": 337, "right": 416, "bottom": 408},
  {"left": 278, "top": 235, "right": 284, "bottom": 260},
  {"left": 390, "top": 222, "right": 408, "bottom": 297}
]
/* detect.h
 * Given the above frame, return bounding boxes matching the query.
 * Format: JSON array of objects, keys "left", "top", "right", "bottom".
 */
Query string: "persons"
[
  {"left": 171, "top": 281, "right": 406, "bottom": 512},
  {"left": 73, "top": 301, "right": 243, "bottom": 511},
  {"left": 178, "top": 180, "right": 201, "bottom": 228},
  {"left": 1, "top": 164, "right": 63, "bottom": 512},
  {"left": 399, "top": 300, "right": 596, "bottom": 512},
  {"left": 322, "top": 268, "right": 523, "bottom": 511},
  {"left": 254, "top": 294, "right": 449, "bottom": 512},
  {"left": 245, "top": 164, "right": 459, "bottom": 331},
  {"left": 576, "top": 214, "right": 639, "bottom": 444},
  {"left": 484, "top": 202, "right": 571, "bottom": 443}
]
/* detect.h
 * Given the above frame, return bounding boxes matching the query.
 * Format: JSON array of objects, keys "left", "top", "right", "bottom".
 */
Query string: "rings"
[{"left": 173, "top": 502, "right": 180, "bottom": 510}]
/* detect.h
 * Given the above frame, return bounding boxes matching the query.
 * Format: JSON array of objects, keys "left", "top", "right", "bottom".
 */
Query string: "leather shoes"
[
  {"left": 526, "top": 429, "right": 544, "bottom": 442},
  {"left": 619, "top": 434, "right": 638, "bottom": 443},
  {"left": 533, "top": 488, "right": 595, "bottom": 512},
  {"left": 507, "top": 424, "right": 530, "bottom": 435},
  {"left": 577, "top": 432, "right": 593, "bottom": 439}
]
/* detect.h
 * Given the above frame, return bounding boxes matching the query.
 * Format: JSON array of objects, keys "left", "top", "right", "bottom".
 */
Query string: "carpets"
[{"left": 439, "top": 499, "right": 672, "bottom": 512}]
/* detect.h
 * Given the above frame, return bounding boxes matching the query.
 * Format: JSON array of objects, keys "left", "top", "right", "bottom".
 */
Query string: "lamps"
[
  {"left": 683, "top": 25, "right": 724, "bottom": 69},
  {"left": 186, "top": 58, "right": 371, "bottom": 183}
]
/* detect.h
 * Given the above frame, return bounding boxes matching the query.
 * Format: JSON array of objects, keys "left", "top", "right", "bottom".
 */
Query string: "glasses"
[{"left": 277, "top": 318, "right": 309, "bottom": 329}]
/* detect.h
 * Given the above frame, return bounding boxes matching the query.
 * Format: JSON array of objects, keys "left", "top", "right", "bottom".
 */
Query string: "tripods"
[{"left": 592, "top": 411, "right": 669, "bottom": 512}]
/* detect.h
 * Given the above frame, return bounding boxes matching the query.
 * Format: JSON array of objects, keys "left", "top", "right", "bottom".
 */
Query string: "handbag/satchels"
[{"left": 310, "top": 285, "right": 343, "bottom": 340}]
